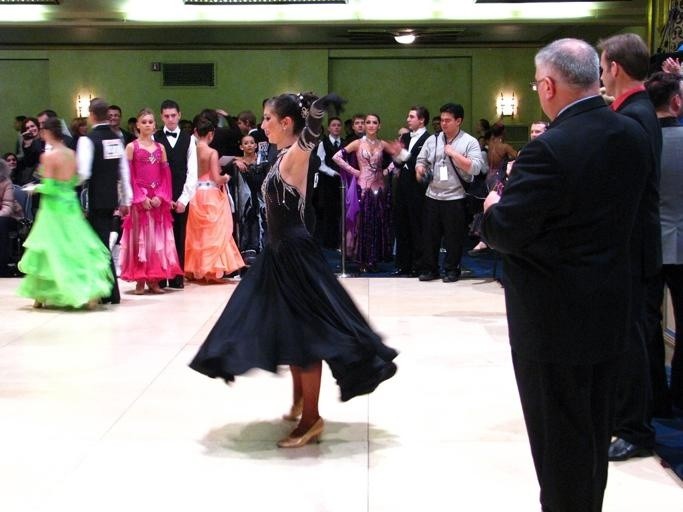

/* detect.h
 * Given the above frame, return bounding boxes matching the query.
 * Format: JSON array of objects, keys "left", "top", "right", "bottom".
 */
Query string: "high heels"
[
  {"left": 146, "top": 280, "right": 165, "bottom": 294},
  {"left": 34, "top": 297, "right": 45, "bottom": 308},
  {"left": 135, "top": 282, "right": 145, "bottom": 294},
  {"left": 277, "top": 416, "right": 323, "bottom": 447},
  {"left": 284, "top": 400, "right": 303, "bottom": 419},
  {"left": 86, "top": 299, "right": 102, "bottom": 310}
]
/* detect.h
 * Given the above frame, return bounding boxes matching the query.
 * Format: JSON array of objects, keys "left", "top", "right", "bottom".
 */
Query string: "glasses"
[{"left": 530, "top": 80, "right": 544, "bottom": 91}]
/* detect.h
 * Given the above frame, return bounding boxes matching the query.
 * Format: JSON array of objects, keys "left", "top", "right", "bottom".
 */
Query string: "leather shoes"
[
  {"left": 419, "top": 270, "right": 439, "bottom": 280},
  {"left": 444, "top": 271, "right": 458, "bottom": 281},
  {"left": 607, "top": 437, "right": 642, "bottom": 460}
]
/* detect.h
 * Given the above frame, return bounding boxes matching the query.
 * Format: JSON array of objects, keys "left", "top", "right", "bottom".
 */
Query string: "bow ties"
[{"left": 166, "top": 131, "right": 177, "bottom": 138}]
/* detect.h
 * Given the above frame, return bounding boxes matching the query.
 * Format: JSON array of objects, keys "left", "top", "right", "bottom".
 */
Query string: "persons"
[
  {"left": 190, "top": 93, "right": 400, "bottom": 450},
  {"left": 313, "top": 102, "right": 484, "bottom": 283},
  {"left": 478, "top": 34, "right": 683, "bottom": 512},
  {"left": 0, "top": 96, "right": 277, "bottom": 310}
]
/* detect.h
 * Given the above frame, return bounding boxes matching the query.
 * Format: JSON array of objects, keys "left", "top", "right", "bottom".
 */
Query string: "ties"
[{"left": 335, "top": 141, "right": 338, "bottom": 148}]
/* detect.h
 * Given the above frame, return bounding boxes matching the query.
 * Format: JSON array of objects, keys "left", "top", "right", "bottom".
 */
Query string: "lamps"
[
  {"left": 496, "top": 89, "right": 518, "bottom": 118},
  {"left": 74, "top": 90, "right": 96, "bottom": 120}
]
